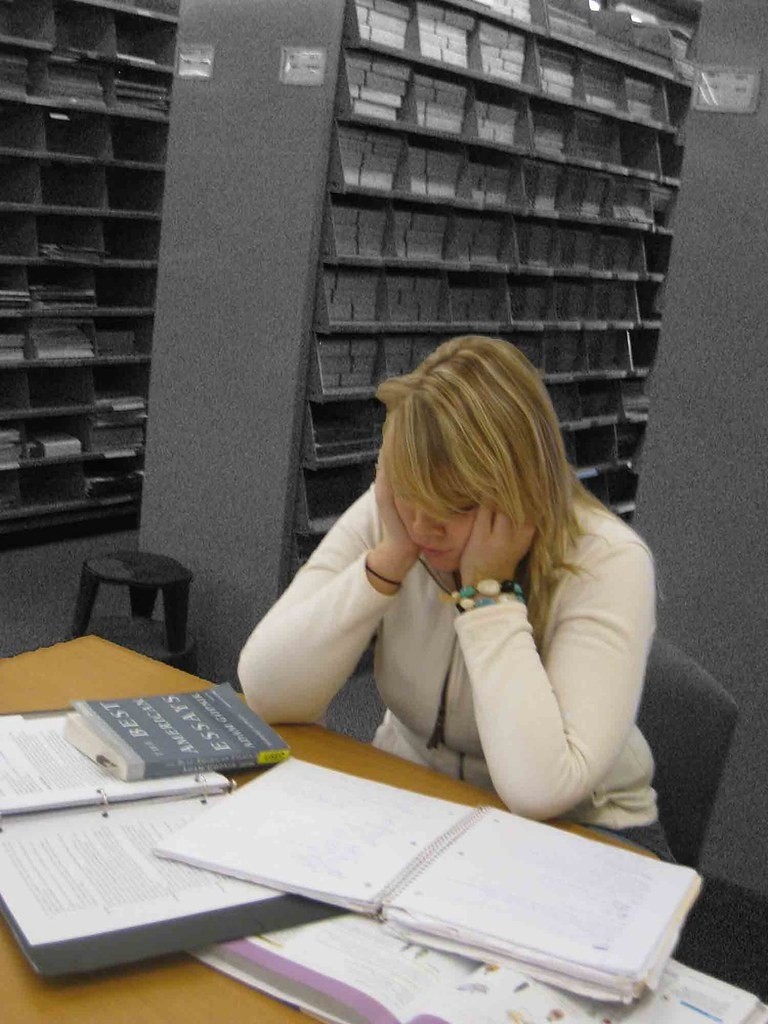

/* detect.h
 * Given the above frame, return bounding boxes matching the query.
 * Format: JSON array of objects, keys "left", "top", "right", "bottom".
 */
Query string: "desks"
[{"left": 0, "top": 632, "right": 659, "bottom": 1024}]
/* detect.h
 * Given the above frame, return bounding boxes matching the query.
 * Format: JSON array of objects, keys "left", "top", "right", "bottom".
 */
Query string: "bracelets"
[
  {"left": 439, "top": 580, "right": 525, "bottom": 609},
  {"left": 366, "top": 564, "right": 403, "bottom": 585}
]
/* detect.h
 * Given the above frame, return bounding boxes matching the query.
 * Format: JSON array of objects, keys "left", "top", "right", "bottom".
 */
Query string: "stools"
[{"left": 72, "top": 551, "right": 195, "bottom": 667}]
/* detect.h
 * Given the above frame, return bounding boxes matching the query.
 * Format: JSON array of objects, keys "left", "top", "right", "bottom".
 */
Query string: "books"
[
  {"left": 0, "top": 0, "right": 699, "bottom": 510},
  {"left": 1, "top": 682, "right": 767, "bottom": 1024}
]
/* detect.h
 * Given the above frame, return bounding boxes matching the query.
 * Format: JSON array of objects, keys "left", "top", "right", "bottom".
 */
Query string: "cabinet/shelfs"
[{"left": 0, "top": 0, "right": 768, "bottom": 897}]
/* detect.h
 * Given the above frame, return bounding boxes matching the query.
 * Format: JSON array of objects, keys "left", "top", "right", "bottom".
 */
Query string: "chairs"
[{"left": 637, "top": 639, "right": 739, "bottom": 868}]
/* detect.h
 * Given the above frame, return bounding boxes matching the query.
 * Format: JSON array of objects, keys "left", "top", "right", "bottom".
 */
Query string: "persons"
[{"left": 236, "top": 335, "right": 677, "bottom": 863}]
